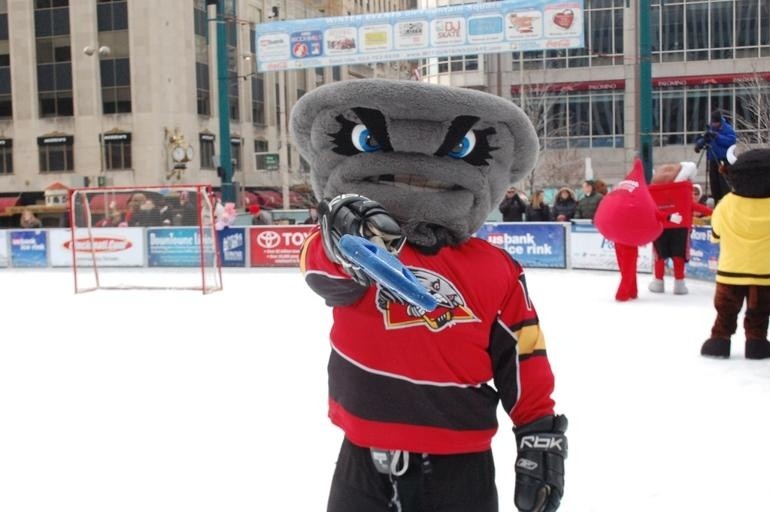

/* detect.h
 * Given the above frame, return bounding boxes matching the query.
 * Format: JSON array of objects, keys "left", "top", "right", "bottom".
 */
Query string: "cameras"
[{"left": 694, "top": 125, "right": 717, "bottom": 152}]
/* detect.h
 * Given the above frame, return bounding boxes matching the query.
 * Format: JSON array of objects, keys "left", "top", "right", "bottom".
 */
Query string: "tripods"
[{"left": 693, "top": 144, "right": 734, "bottom": 204}]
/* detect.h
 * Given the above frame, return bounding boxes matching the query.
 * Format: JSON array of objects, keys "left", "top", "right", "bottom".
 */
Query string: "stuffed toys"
[
  {"left": 701, "top": 146, "right": 770, "bottom": 361},
  {"left": 287, "top": 76, "right": 569, "bottom": 511},
  {"left": 644, "top": 163, "right": 713, "bottom": 295},
  {"left": 594, "top": 160, "right": 683, "bottom": 300}
]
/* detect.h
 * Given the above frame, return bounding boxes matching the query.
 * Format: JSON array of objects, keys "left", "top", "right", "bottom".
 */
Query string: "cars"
[{"left": 230, "top": 208, "right": 314, "bottom": 226}]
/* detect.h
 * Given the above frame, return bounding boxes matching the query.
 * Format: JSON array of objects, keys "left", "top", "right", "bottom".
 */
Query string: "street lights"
[{"left": 83, "top": 38, "right": 110, "bottom": 187}]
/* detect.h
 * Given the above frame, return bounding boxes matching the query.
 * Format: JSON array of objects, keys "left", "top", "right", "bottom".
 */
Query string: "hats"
[{"left": 711, "top": 110, "right": 721, "bottom": 122}]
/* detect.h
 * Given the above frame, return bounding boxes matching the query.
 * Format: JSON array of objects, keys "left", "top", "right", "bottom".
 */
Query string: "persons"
[
  {"left": 99, "top": 192, "right": 227, "bottom": 227},
  {"left": 303, "top": 206, "right": 318, "bottom": 223},
  {"left": 247, "top": 205, "right": 273, "bottom": 224},
  {"left": 694, "top": 110, "right": 735, "bottom": 198},
  {"left": 500, "top": 183, "right": 609, "bottom": 221},
  {"left": 20, "top": 209, "right": 42, "bottom": 229}
]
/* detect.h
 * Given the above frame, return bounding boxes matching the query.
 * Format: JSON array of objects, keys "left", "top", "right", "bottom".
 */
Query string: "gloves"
[
  {"left": 317, "top": 189, "right": 407, "bottom": 292},
  {"left": 510, "top": 415, "right": 570, "bottom": 512}
]
